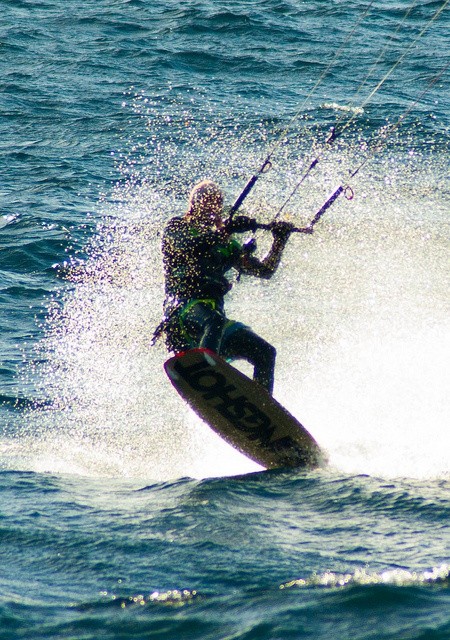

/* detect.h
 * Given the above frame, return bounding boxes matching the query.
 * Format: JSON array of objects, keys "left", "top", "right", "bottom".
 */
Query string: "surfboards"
[{"left": 163, "top": 346, "right": 328, "bottom": 469}]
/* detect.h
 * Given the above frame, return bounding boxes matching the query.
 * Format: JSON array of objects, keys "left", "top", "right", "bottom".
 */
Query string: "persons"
[{"left": 161, "top": 177, "right": 296, "bottom": 397}]
